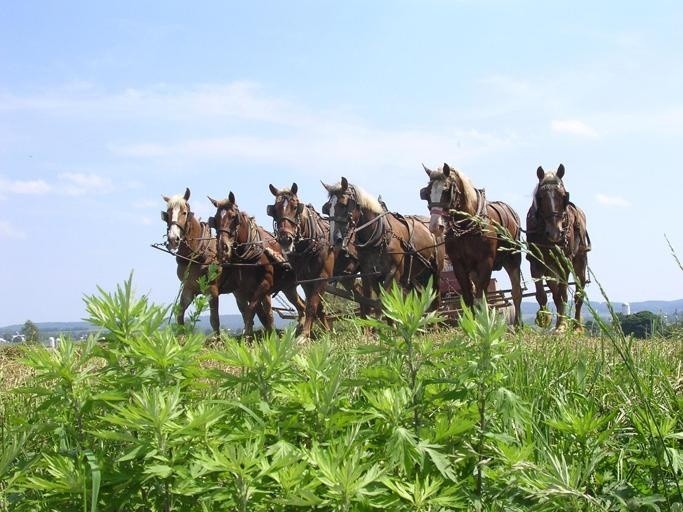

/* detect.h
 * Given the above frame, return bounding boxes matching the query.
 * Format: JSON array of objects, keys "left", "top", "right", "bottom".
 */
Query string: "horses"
[
  {"left": 267, "top": 183, "right": 373, "bottom": 340},
  {"left": 526, "top": 163, "right": 587, "bottom": 336},
  {"left": 421, "top": 164, "right": 521, "bottom": 333},
  {"left": 160, "top": 187, "right": 249, "bottom": 347},
  {"left": 320, "top": 176, "right": 443, "bottom": 334},
  {"left": 206, "top": 192, "right": 306, "bottom": 346}
]
